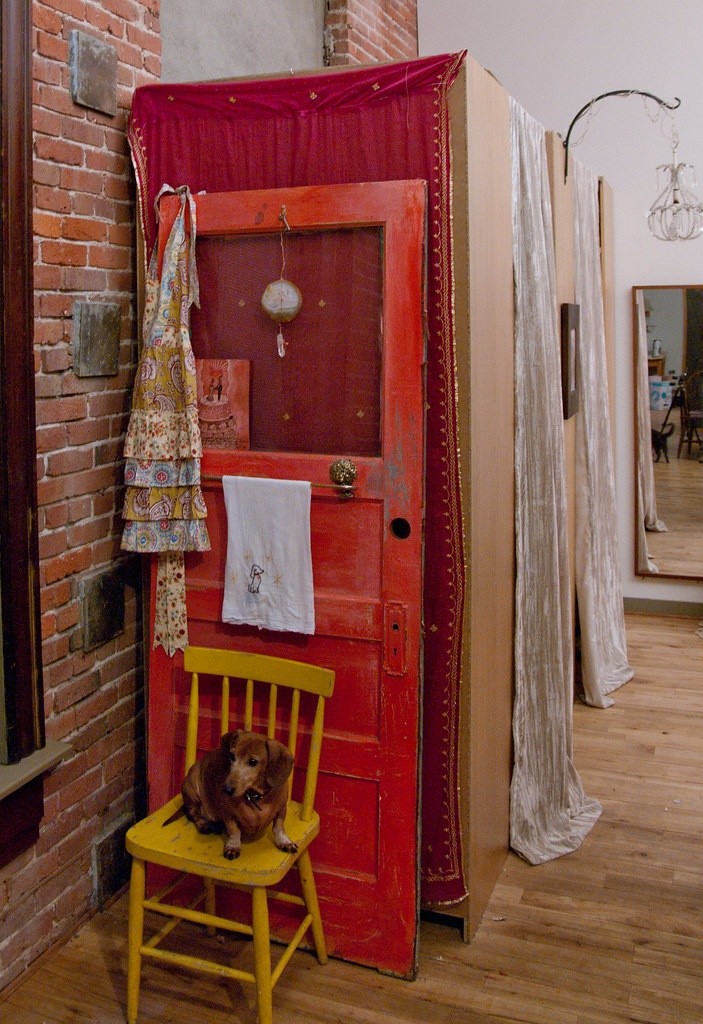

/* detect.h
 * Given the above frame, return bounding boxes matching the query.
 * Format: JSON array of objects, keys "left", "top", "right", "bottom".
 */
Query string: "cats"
[{"left": 651, "top": 422, "right": 676, "bottom": 463}]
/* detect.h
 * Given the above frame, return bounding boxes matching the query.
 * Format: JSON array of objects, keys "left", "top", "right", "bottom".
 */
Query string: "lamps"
[{"left": 564, "top": 90, "right": 703, "bottom": 241}]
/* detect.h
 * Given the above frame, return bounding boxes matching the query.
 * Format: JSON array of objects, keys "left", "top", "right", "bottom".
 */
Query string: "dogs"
[{"left": 162, "top": 728, "right": 301, "bottom": 861}]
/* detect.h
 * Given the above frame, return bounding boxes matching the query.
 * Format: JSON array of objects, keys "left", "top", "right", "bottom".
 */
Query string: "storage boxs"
[{"left": 650, "top": 375, "right": 673, "bottom": 411}]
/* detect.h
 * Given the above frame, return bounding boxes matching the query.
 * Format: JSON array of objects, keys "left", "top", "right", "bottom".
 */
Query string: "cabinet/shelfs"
[{"left": 131, "top": 52, "right": 615, "bottom": 942}]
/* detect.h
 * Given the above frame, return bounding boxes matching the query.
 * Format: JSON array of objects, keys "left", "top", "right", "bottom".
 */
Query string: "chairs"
[
  {"left": 126, "top": 647, "right": 336, "bottom": 1024},
  {"left": 678, "top": 373, "right": 703, "bottom": 460}
]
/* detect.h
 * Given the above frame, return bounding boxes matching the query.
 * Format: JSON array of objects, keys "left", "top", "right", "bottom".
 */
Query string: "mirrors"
[{"left": 631, "top": 284, "right": 703, "bottom": 581}]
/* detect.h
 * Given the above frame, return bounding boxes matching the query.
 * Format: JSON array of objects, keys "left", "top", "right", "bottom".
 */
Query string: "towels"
[{"left": 222, "top": 475, "right": 316, "bottom": 633}]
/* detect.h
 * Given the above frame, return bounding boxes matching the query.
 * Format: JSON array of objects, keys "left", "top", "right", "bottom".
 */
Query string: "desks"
[{"left": 648, "top": 354, "right": 665, "bottom": 375}]
